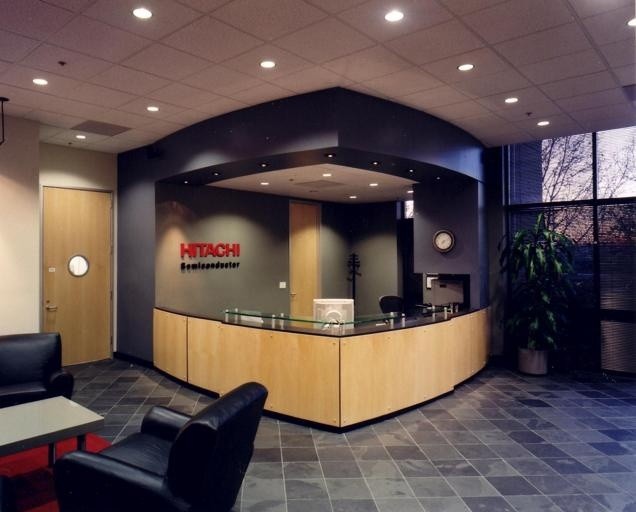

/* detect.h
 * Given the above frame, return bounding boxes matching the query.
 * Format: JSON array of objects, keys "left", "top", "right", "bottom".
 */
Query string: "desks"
[{"left": 1, "top": 400, "right": 109, "bottom": 482}]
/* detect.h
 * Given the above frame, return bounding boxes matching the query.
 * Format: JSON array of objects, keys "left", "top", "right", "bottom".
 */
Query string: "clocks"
[{"left": 433, "top": 230, "right": 455, "bottom": 253}]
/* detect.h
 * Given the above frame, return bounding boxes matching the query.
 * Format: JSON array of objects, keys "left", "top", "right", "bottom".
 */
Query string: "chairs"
[
  {"left": 376, "top": 292, "right": 407, "bottom": 325},
  {"left": 0, "top": 332, "right": 78, "bottom": 396},
  {"left": 55, "top": 380, "right": 268, "bottom": 511}
]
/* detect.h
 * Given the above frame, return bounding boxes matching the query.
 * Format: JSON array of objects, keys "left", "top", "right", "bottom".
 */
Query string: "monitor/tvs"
[{"left": 313, "top": 298, "right": 355, "bottom": 329}]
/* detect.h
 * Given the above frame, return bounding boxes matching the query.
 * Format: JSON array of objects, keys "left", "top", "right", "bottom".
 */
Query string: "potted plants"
[{"left": 493, "top": 207, "right": 584, "bottom": 376}]
